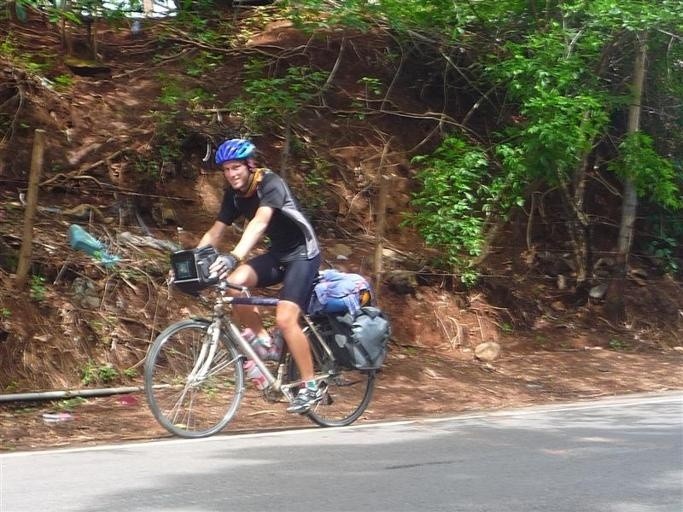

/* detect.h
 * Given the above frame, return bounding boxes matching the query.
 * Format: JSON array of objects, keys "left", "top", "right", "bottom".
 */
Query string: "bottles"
[{"left": 241, "top": 328, "right": 283, "bottom": 390}]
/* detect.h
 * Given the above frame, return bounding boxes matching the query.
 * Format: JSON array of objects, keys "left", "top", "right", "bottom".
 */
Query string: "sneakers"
[
  {"left": 241, "top": 337, "right": 282, "bottom": 361},
  {"left": 286, "top": 386, "right": 323, "bottom": 413}
]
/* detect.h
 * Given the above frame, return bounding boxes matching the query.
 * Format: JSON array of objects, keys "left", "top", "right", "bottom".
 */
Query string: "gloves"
[{"left": 208, "top": 253, "right": 240, "bottom": 281}]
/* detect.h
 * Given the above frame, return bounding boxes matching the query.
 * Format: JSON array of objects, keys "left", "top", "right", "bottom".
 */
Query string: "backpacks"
[
  {"left": 307, "top": 269, "right": 372, "bottom": 314},
  {"left": 329, "top": 306, "right": 390, "bottom": 372}
]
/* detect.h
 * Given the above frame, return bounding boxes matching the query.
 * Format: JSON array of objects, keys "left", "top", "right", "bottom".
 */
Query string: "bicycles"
[{"left": 144, "top": 247, "right": 379, "bottom": 437}]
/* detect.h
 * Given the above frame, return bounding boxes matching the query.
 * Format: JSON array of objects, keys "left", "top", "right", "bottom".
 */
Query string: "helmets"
[{"left": 213, "top": 138, "right": 256, "bottom": 164}]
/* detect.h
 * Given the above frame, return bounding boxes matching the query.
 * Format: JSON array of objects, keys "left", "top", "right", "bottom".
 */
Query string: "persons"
[{"left": 170, "top": 137, "right": 330, "bottom": 413}]
